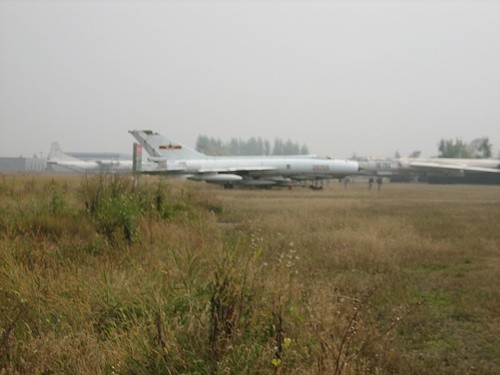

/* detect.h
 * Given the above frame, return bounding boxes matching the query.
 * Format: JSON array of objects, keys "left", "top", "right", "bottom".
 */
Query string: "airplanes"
[{"left": 44, "top": 129, "right": 500, "bottom": 194}]
